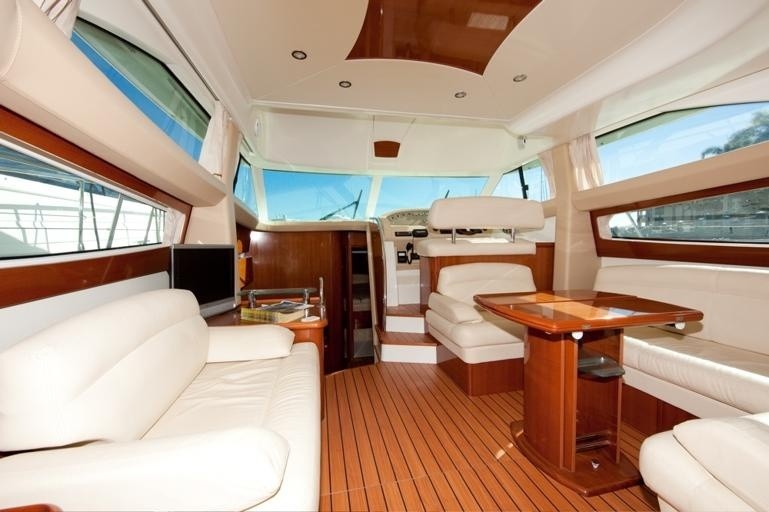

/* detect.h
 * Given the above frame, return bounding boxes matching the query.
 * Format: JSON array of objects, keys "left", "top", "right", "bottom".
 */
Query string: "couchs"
[
  {"left": 0, "top": 271, "right": 321, "bottom": 511},
  {"left": 592, "top": 263, "right": 768, "bottom": 511}
]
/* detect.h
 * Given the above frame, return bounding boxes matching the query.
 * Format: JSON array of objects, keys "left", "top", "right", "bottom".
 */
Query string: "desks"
[{"left": 474, "top": 287, "right": 704, "bottom": 495}]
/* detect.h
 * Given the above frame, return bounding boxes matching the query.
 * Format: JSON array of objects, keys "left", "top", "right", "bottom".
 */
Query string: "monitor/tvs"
[{"left": 171, "top": 244, "right": 241, "bottom": 320}]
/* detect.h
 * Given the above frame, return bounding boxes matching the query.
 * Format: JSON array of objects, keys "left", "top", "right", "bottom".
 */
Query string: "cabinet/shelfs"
[{"left": 205, "top": 297, "right": 327, "bottom": 422}]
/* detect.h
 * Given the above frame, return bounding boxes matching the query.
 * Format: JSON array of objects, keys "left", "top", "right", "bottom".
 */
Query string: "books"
[{"left": 241, "top": 299, "right": 315, "bottom": 325}]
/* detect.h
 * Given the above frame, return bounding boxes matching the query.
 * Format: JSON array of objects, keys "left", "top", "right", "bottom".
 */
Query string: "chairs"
[
  {"left": 415, "top": 195, "right": 553, "bottom": 315},
  {"left": 424, "top": 262, "right": 536, "bottom": 397}
]
